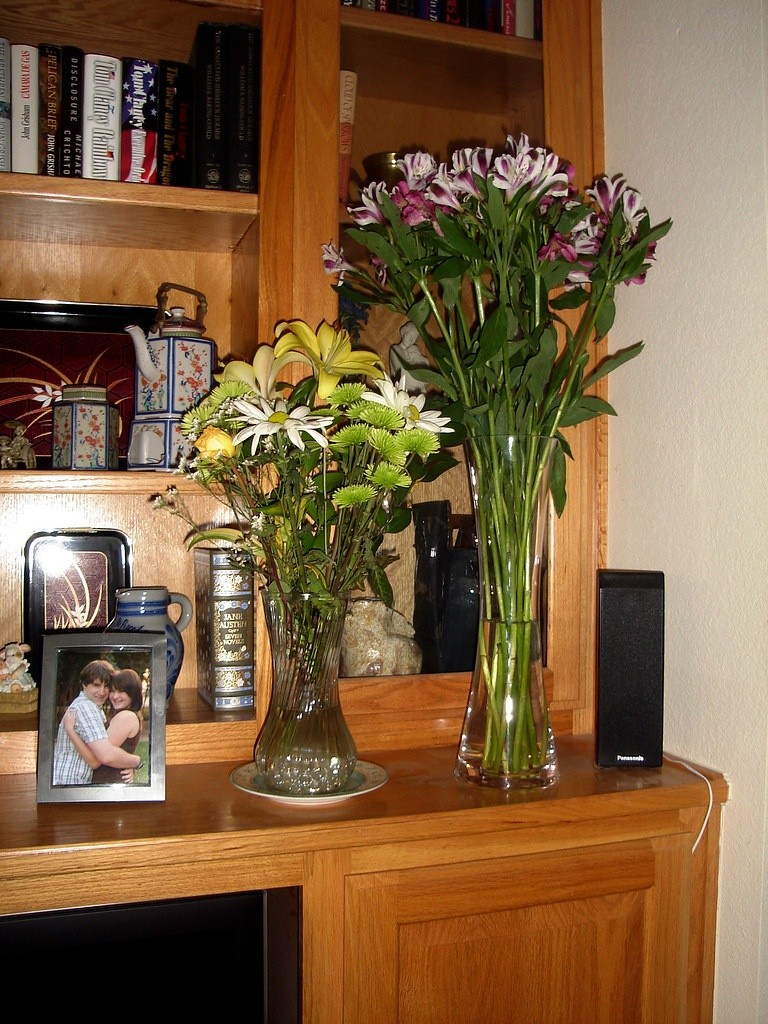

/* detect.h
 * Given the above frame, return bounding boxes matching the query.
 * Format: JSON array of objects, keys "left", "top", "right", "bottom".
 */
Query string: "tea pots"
[{"left": 124, "top": 281, "right": 216, "bottom": 420}]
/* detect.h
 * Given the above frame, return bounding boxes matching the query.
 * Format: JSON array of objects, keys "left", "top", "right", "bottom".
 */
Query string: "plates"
[{"left": 230, "top": 760, "right": 390, "bottom": 804}]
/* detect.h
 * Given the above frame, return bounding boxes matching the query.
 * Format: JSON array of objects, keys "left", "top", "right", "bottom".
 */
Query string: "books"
[
  {"left": 340, "top": 69, "right": 358, "bottom": 205},
  {"left": 0, "top": 18, "right": 258, "bottom": 195},
  {"left": 195, "top": 547, "right": 253, "bottom": 711},
  {"left": 342, "top": 0, "right": 541, "bottom": 41}
]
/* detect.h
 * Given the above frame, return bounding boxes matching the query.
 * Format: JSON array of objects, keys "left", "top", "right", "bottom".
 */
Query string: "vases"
[
  {"left": 256, "top": 590, "right": 356, "bottom": 796},
  {"left": 453, "top": 434, "right": 562, "bottom": 790}
]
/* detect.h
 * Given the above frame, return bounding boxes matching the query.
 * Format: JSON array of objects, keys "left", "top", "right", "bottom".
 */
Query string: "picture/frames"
[{"left": 36, "top": 633, "right": 166, "bottom": 801}]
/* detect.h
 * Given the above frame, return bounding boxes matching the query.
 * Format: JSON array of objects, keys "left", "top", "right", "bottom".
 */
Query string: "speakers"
[{"left": 595, "top": 569, "right": 664, "bottom": 769}]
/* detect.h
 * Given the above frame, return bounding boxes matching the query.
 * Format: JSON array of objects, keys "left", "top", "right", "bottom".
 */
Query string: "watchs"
[{"left": 135, "top": 757, "right": 144, "bottom": 770}]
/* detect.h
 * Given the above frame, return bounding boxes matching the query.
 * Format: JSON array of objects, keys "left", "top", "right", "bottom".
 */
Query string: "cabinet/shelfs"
[{"left": 1, "top": 1, "right": 731, "bottom": 1024}]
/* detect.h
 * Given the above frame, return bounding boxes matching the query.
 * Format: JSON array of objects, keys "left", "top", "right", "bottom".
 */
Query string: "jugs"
[{"left": 103, "top": 587, "right": 192, "bottom": 710}]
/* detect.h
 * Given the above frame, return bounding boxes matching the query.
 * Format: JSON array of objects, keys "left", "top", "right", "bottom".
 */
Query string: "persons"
[
  {"left": 51, "top": 659, "right": 143, "bottom": 785},
  {"left": 390, "top": 321, "right": 429, "bottom": 396}
]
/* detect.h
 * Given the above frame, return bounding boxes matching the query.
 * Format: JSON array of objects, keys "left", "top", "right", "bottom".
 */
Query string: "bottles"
[{"left": 51, "top": 383, "right": 120, "bottom": 470}]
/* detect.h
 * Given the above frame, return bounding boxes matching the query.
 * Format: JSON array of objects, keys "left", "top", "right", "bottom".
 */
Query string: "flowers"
[
  {"left": 154, "top": 320, "right": 466, "bottom": 608},
  {"left": 322, "top": 134, "right": 674, "bottom": 519}
]
